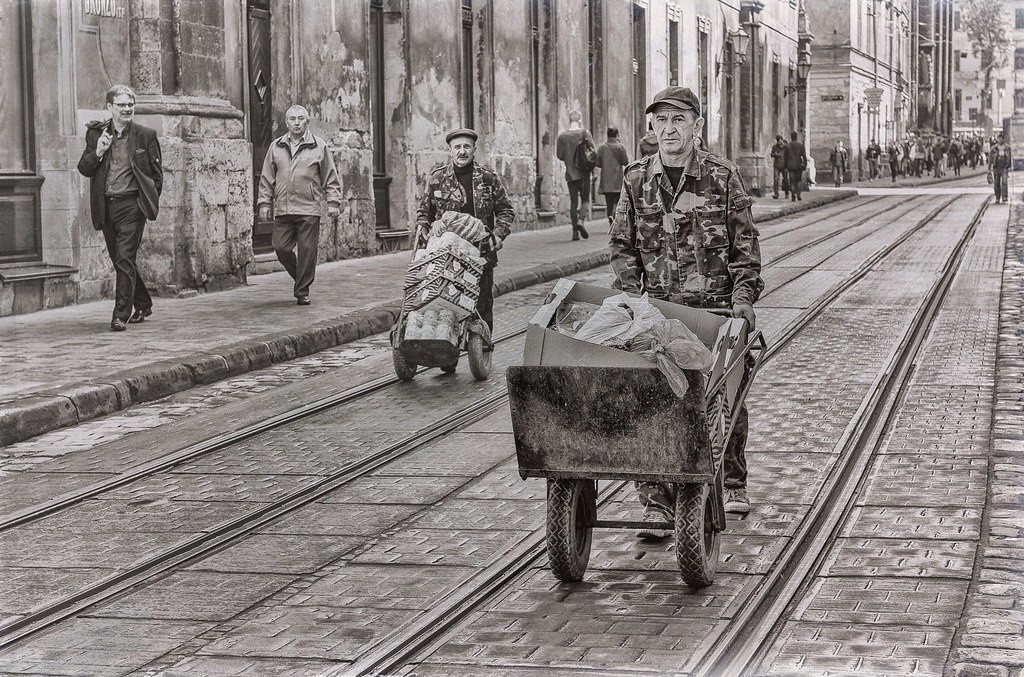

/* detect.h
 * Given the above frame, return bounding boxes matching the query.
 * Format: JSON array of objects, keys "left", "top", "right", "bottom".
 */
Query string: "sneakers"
[
  {"left": 635, "top": 510, "right": 673, "bottom": 539},
  {"left": 723, "top": 487, "right": 751, "bottom": 514}
]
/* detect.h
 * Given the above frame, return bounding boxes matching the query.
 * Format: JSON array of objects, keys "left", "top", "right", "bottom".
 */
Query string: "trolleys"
[
  {"left": 505, "top": 324, "right": 767, "bottom": 587},
  {"left": 390, "top": 219, "right": 495, "bottom": 381}
]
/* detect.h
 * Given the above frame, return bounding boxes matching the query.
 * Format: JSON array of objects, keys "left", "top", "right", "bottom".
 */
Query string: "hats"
[
  {"left": 646, "top": 87, "right": 700, "bottom": 116},
  {"left": 446, "top": 129, "right": 478, "bottom": 144}
]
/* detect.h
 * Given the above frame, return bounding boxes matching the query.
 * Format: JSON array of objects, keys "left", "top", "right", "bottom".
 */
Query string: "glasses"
[{"left": 113, "top": 103, "right": 134, "bottom": 107}]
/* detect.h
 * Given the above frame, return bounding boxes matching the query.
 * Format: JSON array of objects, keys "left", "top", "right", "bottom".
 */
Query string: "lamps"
[
  {"left": 783, "top": 55, "right": 812, "bottom": 96},
  {"left": 715, "top": 19, "right": 750, "bottom": 78}
]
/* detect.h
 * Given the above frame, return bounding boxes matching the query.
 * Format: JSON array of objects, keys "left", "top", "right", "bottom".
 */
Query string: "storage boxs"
[
  {"left": 522, "top": 276, "right": 746, "bottom": 473},
  {"left": 404, "top": 247, "right": 484, "bottom": 322}
]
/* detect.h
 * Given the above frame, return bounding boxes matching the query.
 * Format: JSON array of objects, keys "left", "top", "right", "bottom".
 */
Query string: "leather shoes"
[
  {"left": 110, "top": 318, "right": 126, "bottom": 330},
  {"left": 128, "top": 307, "right": 152, "bottom": 323}
]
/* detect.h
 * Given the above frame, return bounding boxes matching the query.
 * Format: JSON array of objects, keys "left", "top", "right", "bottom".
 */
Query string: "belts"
[{"left": 105, "top": 194, "right": 137, "bottom": 204}]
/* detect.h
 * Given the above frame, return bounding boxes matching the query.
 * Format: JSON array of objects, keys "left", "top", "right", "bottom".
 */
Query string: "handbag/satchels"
[
  {"left": 800, "top": 163, "right": 806, "bottom": 171},
  {"left": 987, "top": 171, "right": 994, "bottom": 185},
  {"left": 572, "top": 129, "right": 597, "bottom": 174}
]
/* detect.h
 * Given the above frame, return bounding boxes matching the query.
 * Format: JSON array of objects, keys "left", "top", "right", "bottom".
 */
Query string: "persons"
[
  {"left": 556, "top": 111, "right": 596, "bottom": 241},
  {"left": 770, "top": 131, "right": 807, "bottom": 201},
  {"left": 636, "top": 121, "right": 659, "bottom": 158},
  {"left": 829, "top": 139, "right": 848, "bottom": 187},
  {"left": 889, "top": 134, "right": 998, "bottom": 182},
  {"left": 988, "top": 135, "right": 1015, "bottom": 203},
  {"left": 416, "top": 128, "right": 516, "bottom": 372},
  {"left": 257, "top": 105, "right": 344, "bottom": 305},
  {"left": 77, "top": 85, "right": 163, "bottom": 332},
  {"left": 597, "top": 126, "right": 628, "bottom": 226},
  {"left": 866, "top": 139, "right": 881, "bottom": 182},
  {"left": 607, "top": 87, "right": 766, "bottom": 536},
  {"left": 693, "top": 137, "right": 709, "bottom": 152}
]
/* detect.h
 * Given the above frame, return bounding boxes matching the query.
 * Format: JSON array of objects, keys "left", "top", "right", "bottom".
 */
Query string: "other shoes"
[
  {"left": 572, "top": 232, "right": 580, "bottom": 241},
  {"left": 792, "top": 196, "right": 796, "bottom": 201},
  {"left": 772, "top": 193, "right": 779, "bottom": 199},
  {"left": 797, "top": 195, "right": 801, "bottom": 200},
  {"left": 867, "top": 165, "right": 977, "bottom": 183},
  {"left": 996, "top": 198, "right": 998, "bottom": 203},
  {"left": 298, "top": 296, "right": 310, "bottom": 306},
  {"left": 609, "top": 215, "right": 615, "bottom": 226},
  {"left": 576, "top": 220, "right": 588, "bottom": 239},
  {"left": 785, "top": 192, "right": 789, "bottom": 199},
  {"left": 1002, "top": 197, "right": 1007, "bottom": 202}
]
